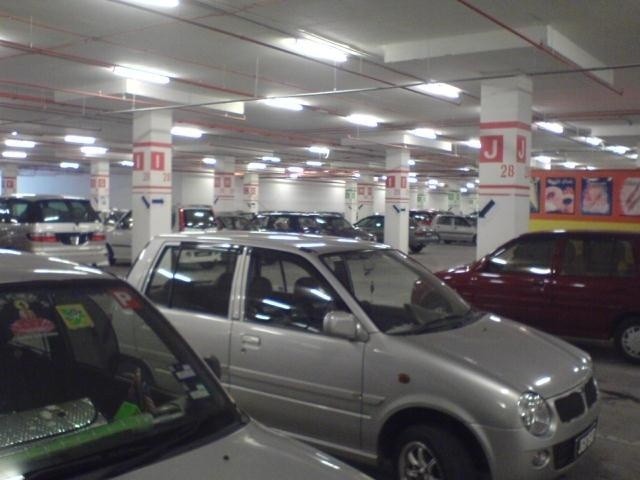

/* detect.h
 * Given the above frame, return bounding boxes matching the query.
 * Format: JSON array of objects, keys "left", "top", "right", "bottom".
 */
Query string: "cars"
[
  {"left": 410, "top": 228, "right": 640, "bottom": 365},
  {"left": 126, "top": 229, "right": 600, "bottom": 480},
  {"left": 0, "top": 247, "right": 373, "bottom": 480}
]
